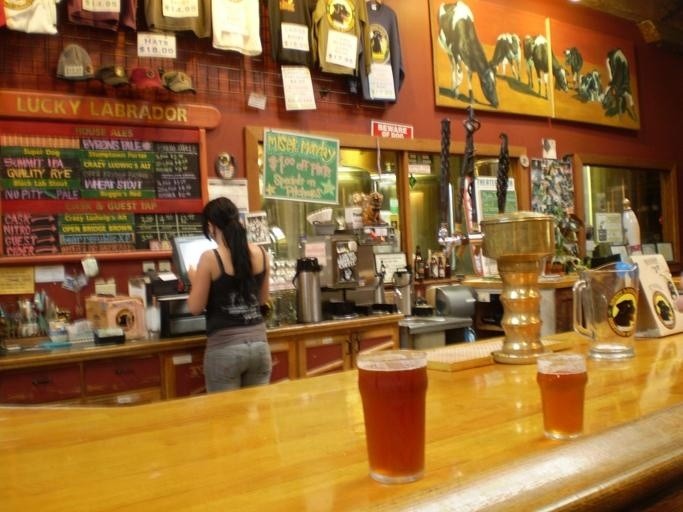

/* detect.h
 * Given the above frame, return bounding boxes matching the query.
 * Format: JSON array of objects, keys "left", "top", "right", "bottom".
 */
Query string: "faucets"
[{"left": 371, "top": 260, "right": 386, "bottom": 303}]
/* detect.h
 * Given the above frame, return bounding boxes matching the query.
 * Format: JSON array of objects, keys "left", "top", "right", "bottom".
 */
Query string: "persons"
[{"left": 188, "top": 197, "right": 272, "bottom": 394}]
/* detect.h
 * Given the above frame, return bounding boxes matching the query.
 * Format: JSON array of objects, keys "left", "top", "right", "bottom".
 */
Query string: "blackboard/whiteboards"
[
  {"left": 0, "top": 111, "right": 209, "bottom": 266},
  {"left": 263, "top": 129, "right": 340, "bottom": 205}
]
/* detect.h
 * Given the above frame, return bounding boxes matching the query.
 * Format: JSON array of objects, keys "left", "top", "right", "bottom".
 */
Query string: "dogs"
[
  {"left": 615, "top": 299, "right": 636, "bottom": 327},
  {"left": 658, "top": 299, "right": 671, "bottom": 322},
  {"left": 371, "top": 30, "right": 383, "bottom": 54},
  {"left": 330, "top": 2, "right": 351, "bottom": 25}
]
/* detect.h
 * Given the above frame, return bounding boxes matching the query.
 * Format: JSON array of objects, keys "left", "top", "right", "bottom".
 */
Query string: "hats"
[
  {"left": 55, "top": 44, "right": 95, "bottom": 82},
  {"left": 129, "top": 66, "right": 166, "bottom": 91},
  {"left": 160, "top": 71, "right": 197, "bottom": 96},
  {"left": 95, "top": 63, "right": 129, "bottom": 87}
]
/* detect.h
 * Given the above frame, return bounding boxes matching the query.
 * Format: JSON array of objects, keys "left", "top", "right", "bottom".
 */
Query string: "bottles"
[
  {"left": 391, "top": 268, "right": 415, "bottom": 317},
  {"left": 414, "top": 245, "right": 450, "bottom": 281},
  {"left": 290, "top": 256, "right": 324, "bottom": 325}
]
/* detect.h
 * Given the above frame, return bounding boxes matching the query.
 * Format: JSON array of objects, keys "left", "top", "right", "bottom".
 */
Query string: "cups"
[
  {"left": 536, "top": 352, "right": 589, "bottom": 440},
  {"left": 572, "top": 261, "right": 641, "bottom": 360},
  {"left": 355, "top": 351, "right": 429, "bottom": 484}
]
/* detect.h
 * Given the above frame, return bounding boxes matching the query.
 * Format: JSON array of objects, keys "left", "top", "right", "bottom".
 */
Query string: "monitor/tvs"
[{"left": 172, "top": 235, "right": 219, "bottom": 286}]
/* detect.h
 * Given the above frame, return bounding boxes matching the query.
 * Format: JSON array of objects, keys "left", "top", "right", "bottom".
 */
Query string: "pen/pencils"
[{"left": 16, "top": 288, "right": 49, "bottom": 335}]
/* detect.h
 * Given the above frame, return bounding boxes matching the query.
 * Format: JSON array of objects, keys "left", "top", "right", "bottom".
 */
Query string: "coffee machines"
[{"left": 301, "top": 234, "right": 381, "bottom": 320}]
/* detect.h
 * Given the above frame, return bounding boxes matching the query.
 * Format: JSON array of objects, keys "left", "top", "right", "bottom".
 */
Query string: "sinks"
[{"left": 397, "top": 316, "right": 473, "bottom": 350}]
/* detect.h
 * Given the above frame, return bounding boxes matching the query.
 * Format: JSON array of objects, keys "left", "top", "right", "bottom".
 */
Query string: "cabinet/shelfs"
[
  {"left": 298, "top": 319, "right": 401, "bottom": 380},
  {"left": 0, "top": 350, "right": 165, "bottom": 408},
  {"left": 165, "top": 337, "right": 298, "bottom": 413}
]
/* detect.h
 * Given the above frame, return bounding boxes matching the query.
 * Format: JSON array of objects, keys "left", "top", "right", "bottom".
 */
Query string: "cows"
[
  {"left": 489, "top": 33, "right": 637, "bottom": 122},
  {"left": 361, "top": 191, "right": 384, "bottom": 225},
  {"left": 436, "top": 0, "right": 500, "bottom": 109}
]
